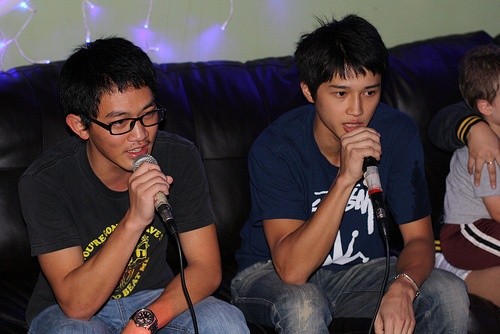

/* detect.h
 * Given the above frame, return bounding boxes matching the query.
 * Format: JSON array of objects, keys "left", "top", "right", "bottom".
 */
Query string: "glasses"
[{"left": 80, "top": 104, "right": 167, "bottom": 135}]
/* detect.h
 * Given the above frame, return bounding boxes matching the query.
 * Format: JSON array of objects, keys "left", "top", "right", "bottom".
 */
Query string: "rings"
[{"left": 489, "top": 162, "right": 495, "bottom": 165}]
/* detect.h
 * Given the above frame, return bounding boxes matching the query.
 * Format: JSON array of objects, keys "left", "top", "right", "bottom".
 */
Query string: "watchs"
[{"left": 129, "top": 308, "right": 158, "bottom": 334}]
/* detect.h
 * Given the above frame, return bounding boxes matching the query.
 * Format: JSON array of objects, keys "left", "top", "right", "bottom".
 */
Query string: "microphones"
[
  {"left": 362, "top": 155, "right": 389, "bottom": 238},
  {"left": 132, "top": 154, "right": 180, "bottom": 238}
]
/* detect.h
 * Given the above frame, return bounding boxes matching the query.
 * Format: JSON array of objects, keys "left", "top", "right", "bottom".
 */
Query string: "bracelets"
[{"left": 393, "top": 274, "right": 420, "bottom": 301}]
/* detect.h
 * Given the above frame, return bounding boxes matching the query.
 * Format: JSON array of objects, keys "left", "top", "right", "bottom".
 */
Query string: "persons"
[
  {"left": 431, "top": 47, "right": 500, "bottom": 308},
  {"left": 441, "top": 50, "right": 500, "bottom": 269},
  {"left": 230, "top": 15, "right": 470, "bottom": 334},
  {"left": 18, "top": 38, "right": 252, "bottom": 334}
]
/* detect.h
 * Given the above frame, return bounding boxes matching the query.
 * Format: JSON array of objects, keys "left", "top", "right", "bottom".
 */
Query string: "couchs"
[{"left": 0, "top": 32, "right": 500, "bottom": 334}]
